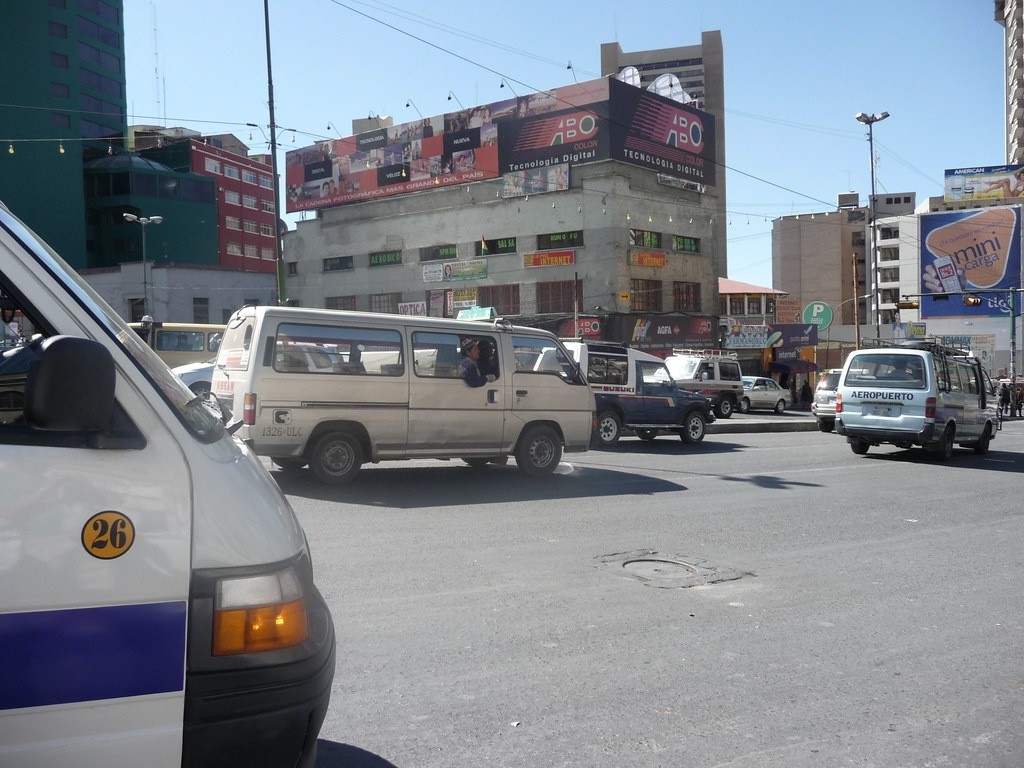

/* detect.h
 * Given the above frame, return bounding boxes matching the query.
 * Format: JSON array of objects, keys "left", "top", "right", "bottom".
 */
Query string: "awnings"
[{"left": 770, "top": 357, "right": 818, "bottom": 373}]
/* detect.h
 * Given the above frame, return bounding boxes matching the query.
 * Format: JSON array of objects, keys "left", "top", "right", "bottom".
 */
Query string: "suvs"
[
  {"left": 812, "top": 370, "right": 875, "bottom": 434},
  {"left": 557, "top": 337, "right": 716, "bottom": 447},
  {"left": 642, "top": 348, "right": 752, "bottom": 416}
]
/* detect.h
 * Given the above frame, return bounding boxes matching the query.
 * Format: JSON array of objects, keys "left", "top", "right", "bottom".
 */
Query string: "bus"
[{"left": 128, "top": 321, "right": 288, "bottom": 372}]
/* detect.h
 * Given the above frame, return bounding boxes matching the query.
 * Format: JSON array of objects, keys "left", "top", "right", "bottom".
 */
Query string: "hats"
[{"left": 460, "top": 338, "right": 479, "bottom": 353}]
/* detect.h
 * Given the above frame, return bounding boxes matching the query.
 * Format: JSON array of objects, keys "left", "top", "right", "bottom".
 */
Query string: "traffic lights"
[{"left": 963, "top": 295, "right": 983, "bottom": 308}]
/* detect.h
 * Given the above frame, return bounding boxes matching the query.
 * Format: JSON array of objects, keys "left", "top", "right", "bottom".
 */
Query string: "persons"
[
  {"left": 978, "top": 166, "right": 1024, "bottom": 197},
  {"left": 402, "top": 142, "right": 410, "bottom": 161},
  {"left": 470, "top": 107, "right": 493, "bottom": 130},
  {"left": 423, "top": 118, "right": 434, "bottom": 138},
  {"left": 445, "top": 265, "right": 451, "bottom": 277},
  {"left": 999, "top": 381, "right": 1024, "bottom": 418},
  {"left": 319, "top": 143, "right": 331, "bottom": 160},
  {"left": 883, "top": 358, "right": 913, "bottom": 378},
  {"left": 322, "top": 179, "right": 337, "bottom": 198},
  {"left": 458, "top": 337, "right": 496, "bottom": 388},
  {"left": 451, "top": 152, "right": 474, "bottom": 172},
  {"left": 174, "top": 335, "right": 193, "bottom": 351},
  {"left": 339, "top": 156, "right": 350, "bottom": 175},
  {"left": 801, "top": 380, "right": 814, "bottom": 409}
]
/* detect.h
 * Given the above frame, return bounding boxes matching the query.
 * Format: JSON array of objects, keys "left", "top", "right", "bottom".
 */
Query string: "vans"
[
  {"left": 216, "top": 303, "right": 596, "bottom": 479},
  {"left": 832, "top": 336, "right": 1003, "bottom": 461}
]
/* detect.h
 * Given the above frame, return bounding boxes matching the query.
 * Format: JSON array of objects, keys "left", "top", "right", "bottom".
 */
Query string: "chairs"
[{"left": 332, "top": 361, "right": 368, "bottom": 374}]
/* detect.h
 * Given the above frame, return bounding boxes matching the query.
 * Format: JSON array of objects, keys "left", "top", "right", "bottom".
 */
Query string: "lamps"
[
  {"left": 448, "top": 91, "right": 464, "bottom": 110},
  {"left": 368, "top": 111, "right": 381, "bottom": 128},
  {"left": 567, "top": 61, "right": 578, "bottom": 82},
  {"left": 406, "top": 99, "right": 424, "bottom": 118},
  {"left": 500, "top": 78, "right": 518, "bottom": 96},
  {"left": 327, "top": 121, "right": 343, "bottom": 142}
]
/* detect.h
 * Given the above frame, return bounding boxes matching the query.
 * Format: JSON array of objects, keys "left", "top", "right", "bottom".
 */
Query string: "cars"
[
  {"left": 969, "top": 377, "right": 999, "bottom": 396},
  {"left": 0, "top": 201, "right": 338, "bottom": 767},
  {"left": 735, "top": 376, "right": 794, "bottom": 413},
  {"left": 168, "top": 342, "right": 345, "bottom": 407}
]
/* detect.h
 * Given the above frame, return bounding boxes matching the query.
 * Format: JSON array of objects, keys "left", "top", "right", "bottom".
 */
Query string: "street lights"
[
  {"left": 824, "top": 293, "right": 874, "bottom": 368},
  {"left": 855, "top": 110, "right": 889, "bottom": 344},
  {"left": 121, "top": 212, "right": 163, "bottom": 320}
]
